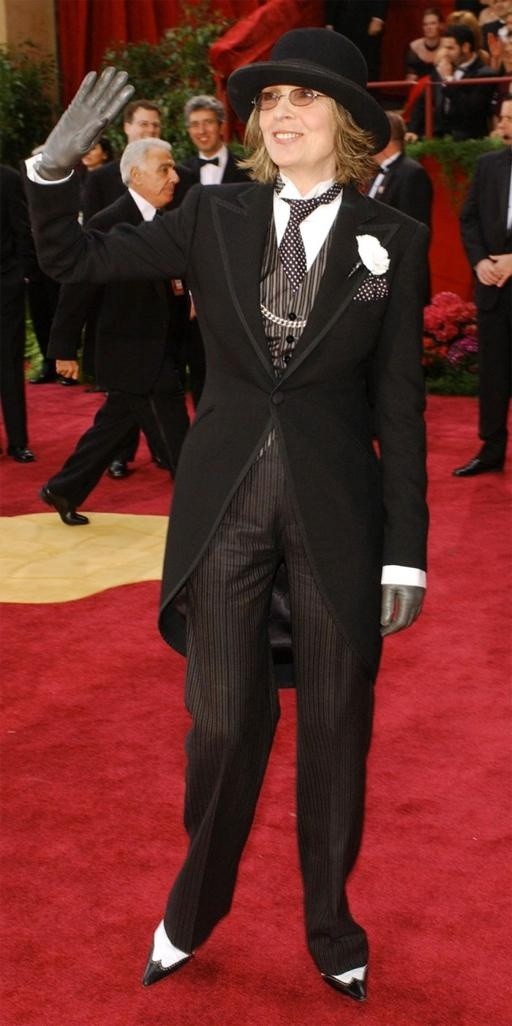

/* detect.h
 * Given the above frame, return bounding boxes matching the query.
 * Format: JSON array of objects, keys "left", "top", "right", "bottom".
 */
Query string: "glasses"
[{"left": 248, "top": 87, "right": 338, "bottom": 113}]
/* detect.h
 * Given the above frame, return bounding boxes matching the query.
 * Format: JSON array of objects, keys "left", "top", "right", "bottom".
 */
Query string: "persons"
[
  {"left": 42, "top": 135, "right": 208, "bottom": 525},
  {"left": 404, "top": 2, "right": 509, "bottom": 142},
  {"left": 82, "top": 98, "right": 163, "bottom": 235},
  {"left": 324, "top": 1, "right": 385, "bottom": 82},
  {"left": 453, "top": 95, "right": 510, "bottom": 481},
  {"left": 30, "top": 30, "right": 428, "bottom": 1001},
  {"left": 2, "top": 145, "right": 36, "bottom": 462},
  {"left": 358, "top": 103, "right": 436, "bottom": 318},
  {"left": 28, "top": 263, "right": 77, "bottom": 384},
  {"left": 171, "top": 88, "right": 258, "bottom": 417},
  {"left": 79, "top": 140, "right": 112, "bottom": 173}
]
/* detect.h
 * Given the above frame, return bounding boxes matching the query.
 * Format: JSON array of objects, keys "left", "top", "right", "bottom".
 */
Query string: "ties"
[{"left": 273, "top": 170, "right": 346, "bottom": 293}]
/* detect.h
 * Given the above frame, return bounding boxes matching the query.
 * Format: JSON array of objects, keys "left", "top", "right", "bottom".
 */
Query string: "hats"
[{"left": 224, "top": 24, "right": 393, "bottom": 156}]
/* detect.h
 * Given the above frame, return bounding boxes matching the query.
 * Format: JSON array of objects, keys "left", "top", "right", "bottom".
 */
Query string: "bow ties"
[
  {"left": 377, "top": 166, "right": 385, "bottom": 173},
  {"left": 196, "top": 156, "right": 221, "bottom": 167},
  {"left": 452, "top": 65, "right": 470, "bottom": 73}
]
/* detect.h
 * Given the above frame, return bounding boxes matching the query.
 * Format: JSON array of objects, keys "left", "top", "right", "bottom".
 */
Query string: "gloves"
[
  {"left": 33, "top": 63, "right": 138, "bottom": 182},
  {"left": 379, "top": 582, "right": 428, "bottom": 637}
]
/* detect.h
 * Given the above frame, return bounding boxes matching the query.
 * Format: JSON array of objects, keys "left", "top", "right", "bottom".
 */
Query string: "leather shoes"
[
  {"left": 27, "top": 366, "right": 80, "bottom": 386},
  {"left": 38, "top": 482, "right": 90, "bottom": 525},
  {"left": 139, "top": 916, "right": 196, "bottom": 987},
  {"left": 107, "top": 458, "right": 130, "bottom": 479},
  {"left": 5, "top": 444, "right": 35, "bottom": 463},
  {"left": 319, "top": 962, "right": 370, "bottom": 1003},
  {"left": 451, "top": 456, "right": 506, "bottom": 477}
]
